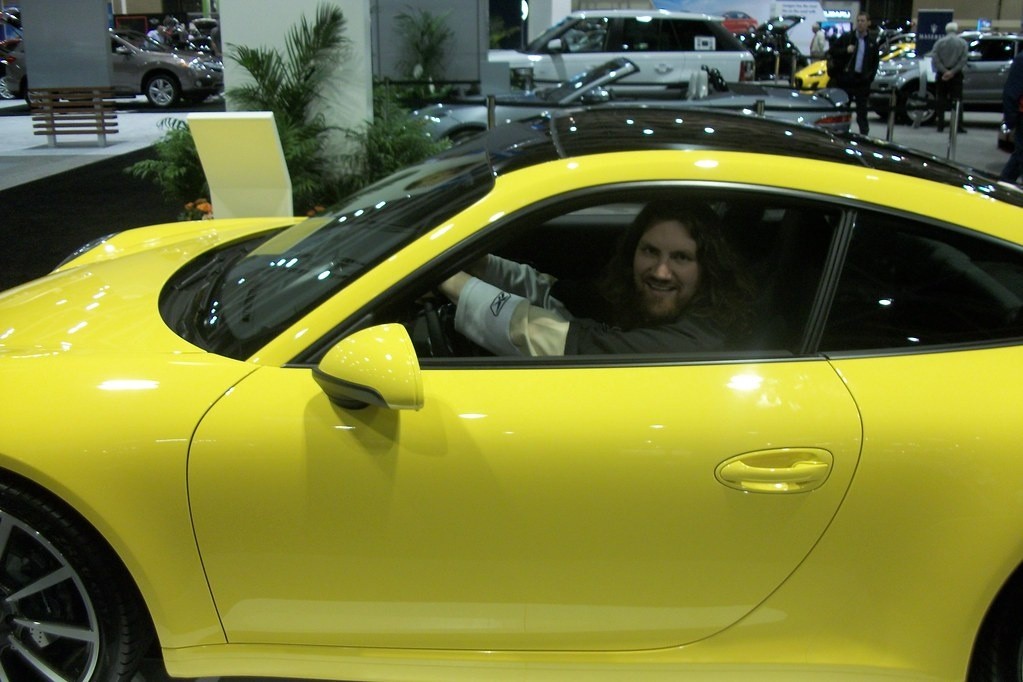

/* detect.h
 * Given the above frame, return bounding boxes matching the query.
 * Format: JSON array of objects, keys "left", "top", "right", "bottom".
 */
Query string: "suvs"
[
  {"left": 487, "top": 10, "right": 756, "bottom": 94},
  {"left": 868, "top": 31, "right": 1023, "bottom": 127}
]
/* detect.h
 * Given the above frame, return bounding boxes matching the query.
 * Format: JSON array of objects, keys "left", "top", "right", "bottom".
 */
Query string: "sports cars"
[{"left": 407, "top": 58, "right": 854, "bottom": 152}]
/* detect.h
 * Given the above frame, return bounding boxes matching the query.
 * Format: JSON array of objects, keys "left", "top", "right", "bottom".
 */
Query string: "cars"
[
  {"left": 113, "top": 12, "right": 223, "bottom": 58},
  {"left": 0, "top": 21, "right": 24, "bottom": 100},
  {"left": 722, "top": 10, "right": 759, "bottom": 35},
  {"left": 0, "top": 108, "right": 1023, "bottom": 682},
  {"left": 717, "top": 14, "right": 915, "bottom": 92},
  {"left": 4, "top": 27, "right": 224, "bottom": 109}
]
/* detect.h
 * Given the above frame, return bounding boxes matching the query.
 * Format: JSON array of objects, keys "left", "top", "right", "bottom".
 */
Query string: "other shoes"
[{"left": 957, "top": 129, "right": 969, "bottom": 134}]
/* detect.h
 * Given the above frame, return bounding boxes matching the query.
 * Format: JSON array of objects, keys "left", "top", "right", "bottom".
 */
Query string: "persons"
[
  {"left": 810, "top": 23, "right": 825, "bottom": 64},
  {"left": 931, "top": 22, "right": 968, "bottom": 133},
  {"left": 436, "top": 201, "right": 754, "bottom": 357},
  {"left": 144, "top": 17, "right": 173, "bottom": 48},
  {"left": 829, "top": 12, "right": 879, "bottom": 136},
  {"left": 999, "top": 49, "right": 1023, "bottom": 181}
]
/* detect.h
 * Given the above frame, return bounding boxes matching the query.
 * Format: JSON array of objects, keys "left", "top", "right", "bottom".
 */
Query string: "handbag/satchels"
[
  {"left": 826, "top": 33, "right": 856, "bottom": 77},
  {"left": 998, "top": 117, "right": 1022, "bottom": 154}
]
